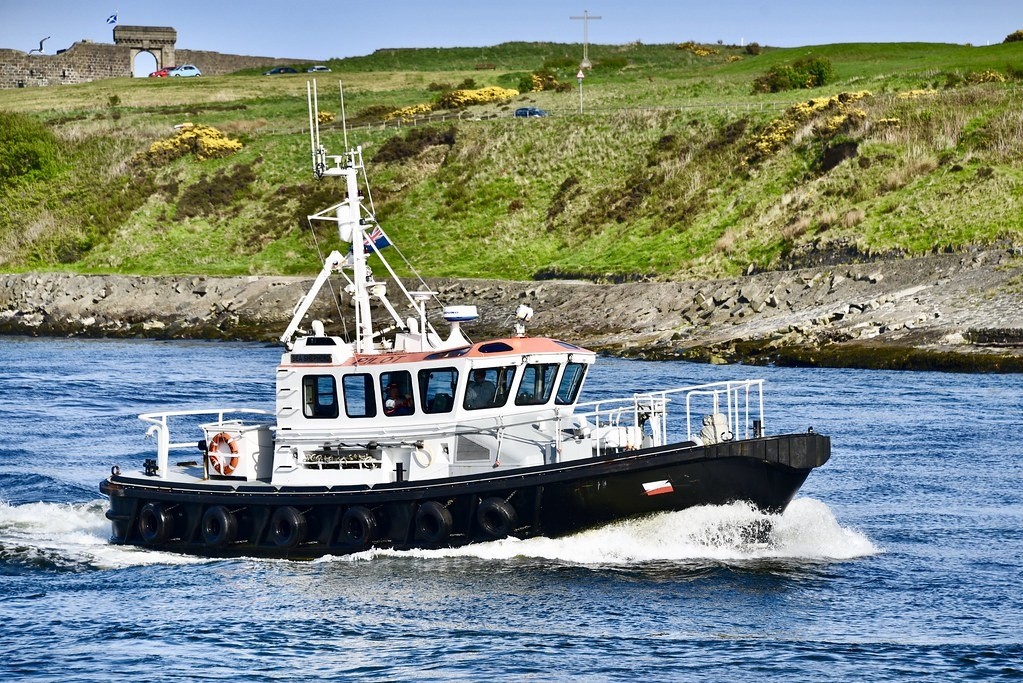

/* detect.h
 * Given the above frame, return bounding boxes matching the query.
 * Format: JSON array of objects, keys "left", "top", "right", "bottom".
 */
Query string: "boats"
[{"left": 98, "top": 71, "right": 832, "bottom": 560}]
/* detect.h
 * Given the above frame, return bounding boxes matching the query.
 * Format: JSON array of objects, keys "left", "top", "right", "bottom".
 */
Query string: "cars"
[
  {"left": 149, "top": 66, "right": 177, "bottom": 78},
  {"left": 308, "top": 66, "right": 332, "bottom": 73},
  {"left": 514, "top": 108, "right": 548, "bottom": 118},
  {"left": 167, "top": 65, "right": 201, "bottom": 77},
  {"left": 263, "top": 67, "right": 298, "bottom": 76}
]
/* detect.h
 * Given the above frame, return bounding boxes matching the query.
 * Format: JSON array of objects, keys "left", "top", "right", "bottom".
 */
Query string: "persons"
[
  {"left": 466, "top": 370, "right": 496, "bottom": 409},
  {"left": 382, "top": 381, "right": 414, "bottom": 415}
]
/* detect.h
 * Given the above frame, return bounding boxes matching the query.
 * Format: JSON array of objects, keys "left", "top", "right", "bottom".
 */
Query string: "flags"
[
  {"left": 349, "top": 225, "right": 391, "bottom": 255},
  {"left": 106, "top": 14, "right": 117, "bottom": 24}
]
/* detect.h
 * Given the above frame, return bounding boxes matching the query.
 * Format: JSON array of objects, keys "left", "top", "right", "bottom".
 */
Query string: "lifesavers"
[
  {"left": 413, "top": 500, "right": 454, "bottom": 545},
  {"left": 200, "top": 503, "right": 238, "bottom": 549},
  {"left": 208, "top": 432, "right": 239, "bottom": 476},
  {"left": 137, "top": 500, "right": 176, "bottom": 546},
  {"left": 338, "top": 504, "right": 378, "bottom": 549},
  {"left": 474, "top": 497, "right": 517, "bottom": 539},
  {"left": 267, "top": 504, "right": 309, "bottom": 549}
]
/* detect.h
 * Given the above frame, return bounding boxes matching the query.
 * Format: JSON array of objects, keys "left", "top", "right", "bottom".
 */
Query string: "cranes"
[{"left": 568, "top": 9, "right": 603, "bottom": 72}]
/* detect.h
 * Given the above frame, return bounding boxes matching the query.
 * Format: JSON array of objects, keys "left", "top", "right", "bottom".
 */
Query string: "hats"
[{"left": 387, "top": 382, "right": 398, "bottom": 393}]
